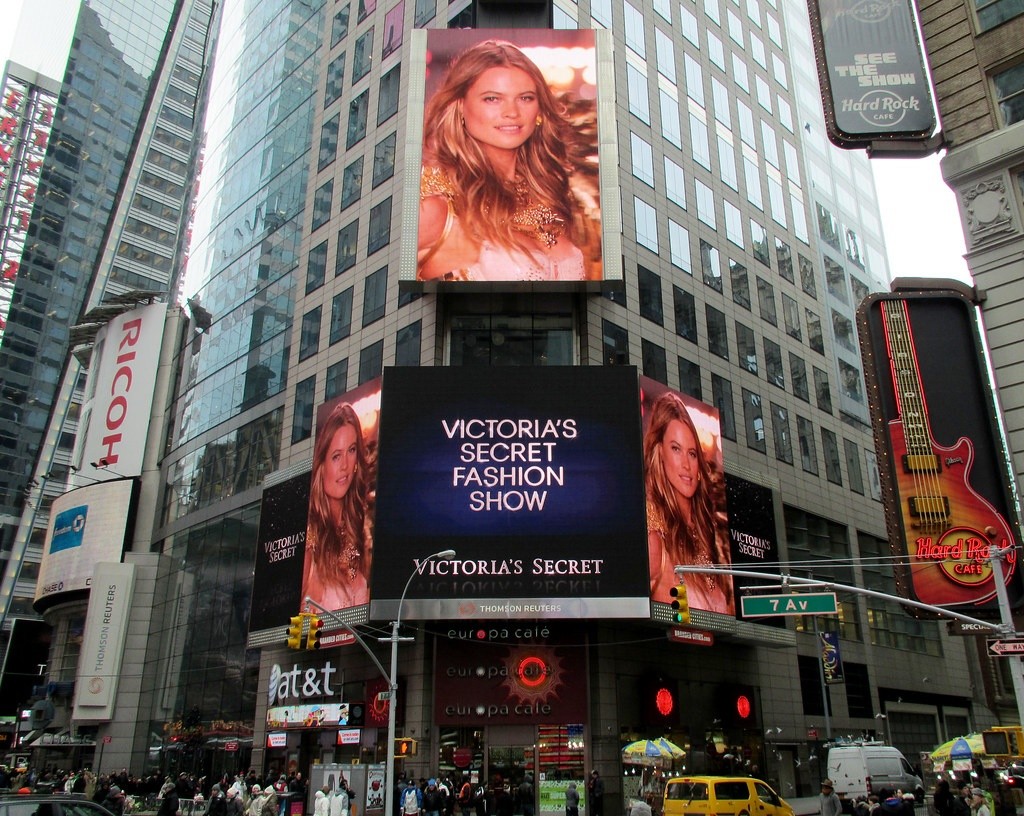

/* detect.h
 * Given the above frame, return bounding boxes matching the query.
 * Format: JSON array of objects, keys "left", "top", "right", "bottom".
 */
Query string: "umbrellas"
[
  {"left": 929, "top": 732, "right": 993, "bottom": 784},
  {"left": 621, "top": 736, "right": 687, "bottom": 759}
]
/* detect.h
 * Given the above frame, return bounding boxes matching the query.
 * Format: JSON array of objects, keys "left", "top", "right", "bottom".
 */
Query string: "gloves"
[{"left": 251, "top": 794, "right": 256, "bottom": 800}]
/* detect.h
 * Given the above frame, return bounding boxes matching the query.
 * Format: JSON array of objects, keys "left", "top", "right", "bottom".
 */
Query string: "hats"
[
  {"left": 110, "top": 785, "right": 120, "bottom": 794},
  {"left": 165, "top": 783, "right": 175, "bottom": 788},
  {"left": 265, "top": 785, "right": 275, "bottom": 793},
  {"left": 970, "top": 787, "right": 984, "bottom": 796},
  {"left": 227, "top": 788, "right": 239, "bottom": 798},
  {"left": 408, "top": 780, "right": 415, "bottom": 787},
  {"left": 253, "top": 784, "right": 260, "bottom": 790},
  {"left": 280, "top": 774, "right": 286, "bottom": 780},
  {"left": 821, "top": 779, "right": 834, "bottom": 789},
  {"left": 428, "top": 778, "right": 436, "bottom": 786},
  {"left": 212, "top": 784, "right": 220, "bottom": 791}
]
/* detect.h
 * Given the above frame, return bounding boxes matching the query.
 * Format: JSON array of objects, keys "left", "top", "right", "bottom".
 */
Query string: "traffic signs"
[{"left": 984, "top": 638, "right": 1024, "bottom": 657}]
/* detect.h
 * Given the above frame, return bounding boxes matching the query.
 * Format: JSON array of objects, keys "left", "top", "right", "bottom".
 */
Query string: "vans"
[
  {"left": 827, "top": 743, "right": 925, "bottom": 804},
  {"left": 660, "top": 775, "right": 796, "bottom": 816}
]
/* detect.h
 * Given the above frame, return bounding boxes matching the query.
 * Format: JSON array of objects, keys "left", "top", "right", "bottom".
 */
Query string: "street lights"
[{"left": 384, "top": 550, "right": 456, "bottom": 816}]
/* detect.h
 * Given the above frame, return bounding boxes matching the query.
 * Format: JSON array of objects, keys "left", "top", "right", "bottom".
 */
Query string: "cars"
[
  {"left": 0, "top": 786, "right": 119, "bottom": 816},
  {"left": 921, "top": 756, "right": 1024, "bottom": 792}
]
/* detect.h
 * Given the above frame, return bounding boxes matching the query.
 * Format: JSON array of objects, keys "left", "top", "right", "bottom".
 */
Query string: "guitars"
[{"left": 877, "top": 299, "right": 1016, "bottom": 610}]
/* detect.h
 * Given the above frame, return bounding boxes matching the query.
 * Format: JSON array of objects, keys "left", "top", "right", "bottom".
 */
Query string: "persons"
[
  {"left": 290, "top": 760, "right": 296, "bottom": 772},
  {"left": 643, "top": 392, "right": 731, "bottom": 615},
  {"left": 303, "top": 402, "right": 377, "bottom": 614},
  {"left": 590, "top": 770, "right": 603, "bottom": 816},
  {"left": 565, "top": 782, "right": 580, "bottom": 816},
  {"left": 394, "top": 771, "right": 534, "bottom": 816},
  {"left": 631, "top": 786, "right": 654, "bottom": 816},
  {"left": 313, "top": 780, "right": 355, "bottom": 816},
  {"left": 0, "top": 768, "right": 309, "bottom": 816},
  {"left": 851, "top": 788, "right": 915, "bottom": 816},
  {"left": 933, "top": 780, "right": 995, "bottom": 816},
  {"left": 820, "top": 778, "right": 842, "bottom": 816},
  {"left": 417, "top": 38, "right": 596, "bottom": 282}
]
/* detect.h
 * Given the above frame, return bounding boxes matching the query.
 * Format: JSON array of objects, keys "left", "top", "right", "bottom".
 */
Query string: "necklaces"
[
  {"left": 683, "top": 524, "right": 716, "bottom": 590},
  {"left": 333, "top": 522, "right": 360, "bottom": 583},
  {"left": 500, "top": 176, "right": 564, "bottom": 249}
]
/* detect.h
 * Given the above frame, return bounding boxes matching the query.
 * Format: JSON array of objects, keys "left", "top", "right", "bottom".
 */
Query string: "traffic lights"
[
  {"left": 284, "top": 617, "right": 305, "bottom": 648},
  {"left": 399, "top": 737, "right": 417, "bottom": 758},
  {"left": 670, "top": 583, "right": 692, "bottom": 626},
  {"left": 305, "top": 617, "right": 325, "bottom": 651},
  {"left": 983, "top": 730, "right": 1013, "bottom": 757}
]
